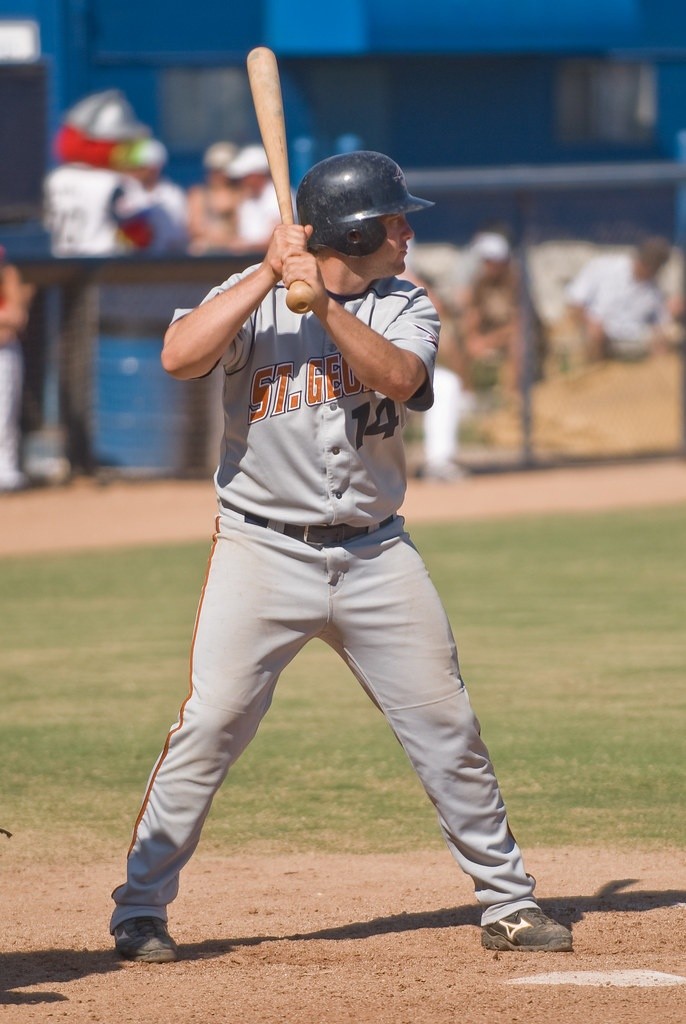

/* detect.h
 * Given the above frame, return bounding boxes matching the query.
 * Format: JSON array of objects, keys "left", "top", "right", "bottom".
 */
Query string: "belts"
[{"left": 244, "top": 514, "right": 393, "bottom": 545}]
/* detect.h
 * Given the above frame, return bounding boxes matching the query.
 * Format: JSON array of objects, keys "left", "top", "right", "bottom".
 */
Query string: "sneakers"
[
  {"left": 481, "top": 908, "right": 573, "bottom": 952},
  {"left": 115, "top": 917, "right": 179, "bottom": 962}
]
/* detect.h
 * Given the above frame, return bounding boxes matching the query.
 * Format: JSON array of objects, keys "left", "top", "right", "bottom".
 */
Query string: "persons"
[
  {"left": 0, "top": 90, "right": 686, "bottom": 492},
  {"left": 107, "top": 151, "right": 573, "bottom": 965}
]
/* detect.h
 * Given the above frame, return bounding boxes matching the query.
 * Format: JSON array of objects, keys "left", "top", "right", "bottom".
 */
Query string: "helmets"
[{"left": 297, "top": 150, "right": 435, "bottom": 258}]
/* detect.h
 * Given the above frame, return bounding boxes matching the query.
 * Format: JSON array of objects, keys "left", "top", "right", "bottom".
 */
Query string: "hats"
[
  {"left": 223, "top": 146, "right": 271, "bottom": 179},
  {"left": 470, "top": 233, "right": 509, "bottom": 264},
  {"left": 128, "top": 138, "right": 167, "bottom": 167},
  {"left": 204, "top": 142, "right": 237, "bottom": 168}
]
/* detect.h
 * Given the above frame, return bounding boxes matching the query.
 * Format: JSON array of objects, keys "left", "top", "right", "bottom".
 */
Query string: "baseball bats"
[{"left": 247, "top": 46, "right": 321, "bottom": 314}]
